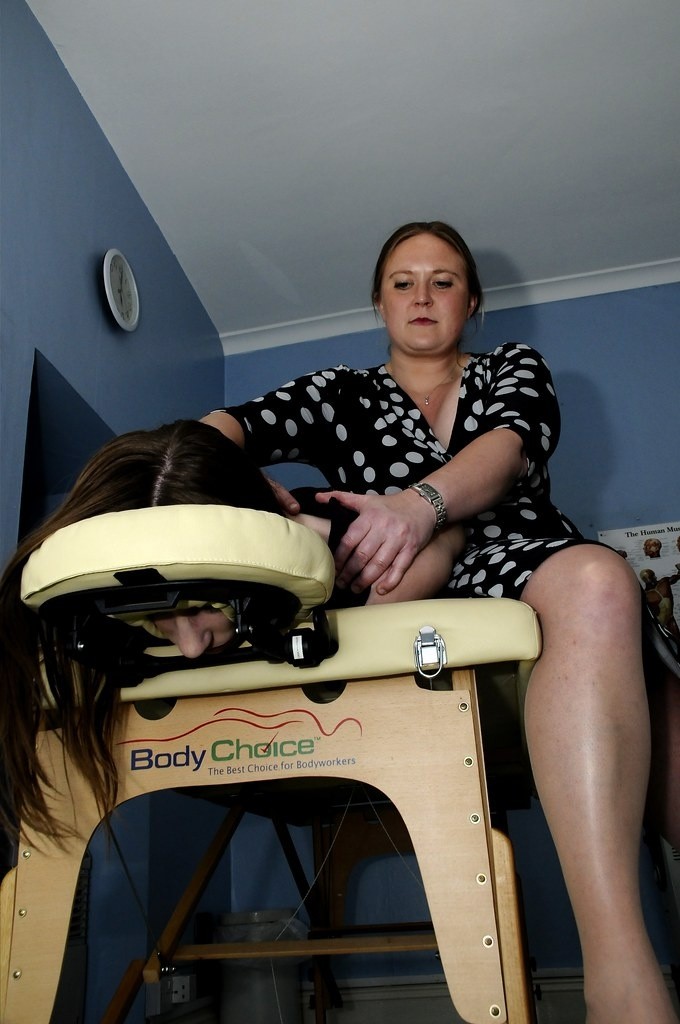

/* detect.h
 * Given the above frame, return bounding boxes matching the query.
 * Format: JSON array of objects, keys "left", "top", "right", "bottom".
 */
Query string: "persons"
[
  {"left": 196, "top": 222, "right": 680, "bottom": 1024},
  {"left": 0, "top": 421, "right": 465, "bottom": 847}
]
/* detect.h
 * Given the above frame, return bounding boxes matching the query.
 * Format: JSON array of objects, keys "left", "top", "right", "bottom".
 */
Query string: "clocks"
[{"left": 101, "top": 248, "right": 141, "bottom": 333}]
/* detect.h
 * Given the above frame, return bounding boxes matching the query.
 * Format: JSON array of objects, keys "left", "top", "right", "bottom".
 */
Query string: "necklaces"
[{"left": 424, "top": 364, "right": 457, "bottom": 404}]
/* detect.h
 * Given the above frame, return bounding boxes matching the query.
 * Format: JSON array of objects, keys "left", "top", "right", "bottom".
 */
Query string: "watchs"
[{"left": 408, "top": 483, "right": 447, "bottom": 528}]
